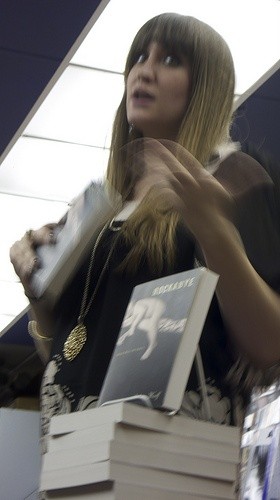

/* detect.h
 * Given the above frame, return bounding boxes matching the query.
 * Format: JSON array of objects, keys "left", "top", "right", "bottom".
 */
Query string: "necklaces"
[{"left": 63, "top": 216, "right": 128, "bottom": 360}]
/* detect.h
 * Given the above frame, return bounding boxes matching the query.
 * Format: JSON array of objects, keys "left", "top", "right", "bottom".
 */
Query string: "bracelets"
[{"left": 26, "top": 321, "right": 53, "bottom": 342}]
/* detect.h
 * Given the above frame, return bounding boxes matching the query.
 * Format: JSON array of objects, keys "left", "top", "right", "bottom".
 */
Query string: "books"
[
  {"left": 39, "top": 401, "right": 244, "bottom": 500},
  {"left": 98, "top": 266, "right": 220, "bottom": 416}
]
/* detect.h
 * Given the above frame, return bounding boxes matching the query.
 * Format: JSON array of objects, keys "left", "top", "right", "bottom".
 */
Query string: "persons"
[{"left": 10, "top": 13, "right": 280, "bottom": 454}]
